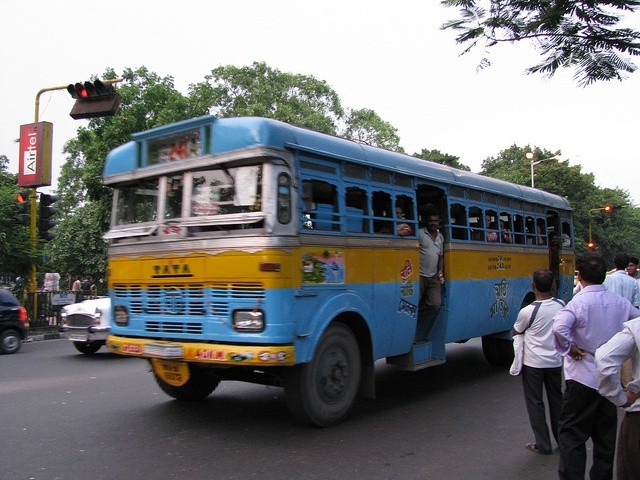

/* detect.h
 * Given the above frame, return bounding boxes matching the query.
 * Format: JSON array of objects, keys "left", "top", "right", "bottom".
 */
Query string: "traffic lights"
[
  {"left": 604, "top": 205, "right": 624, "bottom": 213},
  {"left": 587, "top": 242, "right": 595, "bottom": 250},
  {"left": 66, "top": 77, "right": 124, "bottom": 118}
]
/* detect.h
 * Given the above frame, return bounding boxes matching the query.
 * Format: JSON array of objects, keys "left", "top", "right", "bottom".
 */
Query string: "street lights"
[{"left": 525, "top": 151, "right": 560, "bottom": 188}]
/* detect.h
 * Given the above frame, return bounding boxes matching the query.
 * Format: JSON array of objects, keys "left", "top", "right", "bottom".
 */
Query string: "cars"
[
  {"left": 0, "top": 287, "right": 29, "bottom": 354},
  {"left": 61, "top": 295, "right": 109, "bottom": 353}
]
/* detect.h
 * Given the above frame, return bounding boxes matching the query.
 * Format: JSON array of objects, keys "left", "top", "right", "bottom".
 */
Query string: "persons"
[
  {"left": 573, "top": 282, "right": 583, "bottom": 294},
  {"left": 562, "top": 226, "right": 570, "bottom": 247},
  {"left": 549, "top": 232, "right": 559, "bottom": 291},
  {"left": 72, "top": 276, "right": 83, "bottom": 299},
  {"left": 82, "top": 275, "right": 94, "bottom": 295},
  {"left": 553, "top": 254, "right": 640, "bottom": 479},
  {"left": 626, "top": 257, "right": 640, "bottom": 280},
  {"left": 474, "top": 213, "right": 498, "bottom": 241},
  {"left": 415, "top": 214, "right": 445, "bottom": 344},
  {"left": 496, "top": 220, "right": 512, "bottom": 243},
  {"left": 537, "top": 226, "right": 544, "bottom": 245},
  {"left": 525, "top": 227, "right": 536, "bottom": 244},
  {"left": 510, "top": 269, "right": 566, "bottom": 453},
  {"left": 377, "top": 207, "right": 414, "bottom": 235},
  {"left": 603, "top": 254, "right": 640, "bottom": 307},
  {"left": 595, "top": 315, "right": 639, "bottom": 478}
]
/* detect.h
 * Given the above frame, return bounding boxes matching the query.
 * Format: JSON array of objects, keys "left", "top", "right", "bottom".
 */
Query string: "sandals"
[{"left": 525, "top": 442, "right": 539, "bottom": 452}]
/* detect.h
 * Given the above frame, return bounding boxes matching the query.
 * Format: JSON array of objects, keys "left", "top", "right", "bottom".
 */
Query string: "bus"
[{"left": 104, "top": 115, "right": 575, "bottom": 427}]
